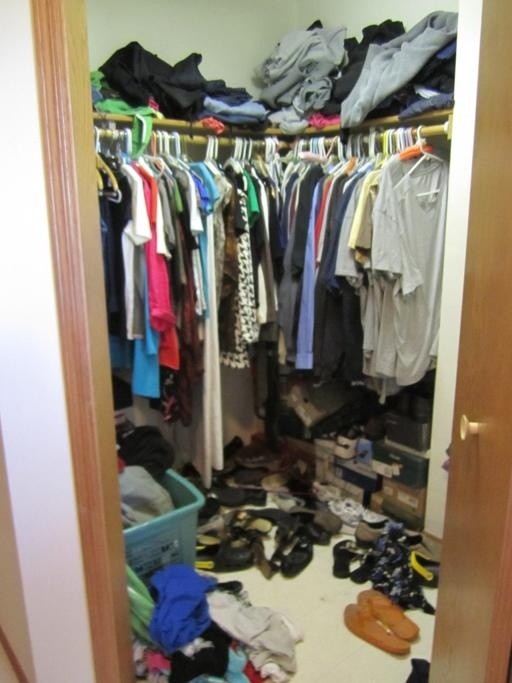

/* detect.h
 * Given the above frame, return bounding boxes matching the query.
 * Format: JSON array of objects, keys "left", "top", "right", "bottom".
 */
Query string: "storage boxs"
[{"left": 264, "top": 357, "right": 439, "bottom": 538}]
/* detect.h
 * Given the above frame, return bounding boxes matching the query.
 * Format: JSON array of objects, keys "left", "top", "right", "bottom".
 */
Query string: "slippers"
[{"left": 344, "top": 589, "right": 419, "bottom": 654}]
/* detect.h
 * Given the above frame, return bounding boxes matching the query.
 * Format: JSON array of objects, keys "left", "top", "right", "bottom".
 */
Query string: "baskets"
[{"left": 119, "top": 467, "right": 206, "bottom": 595}]
